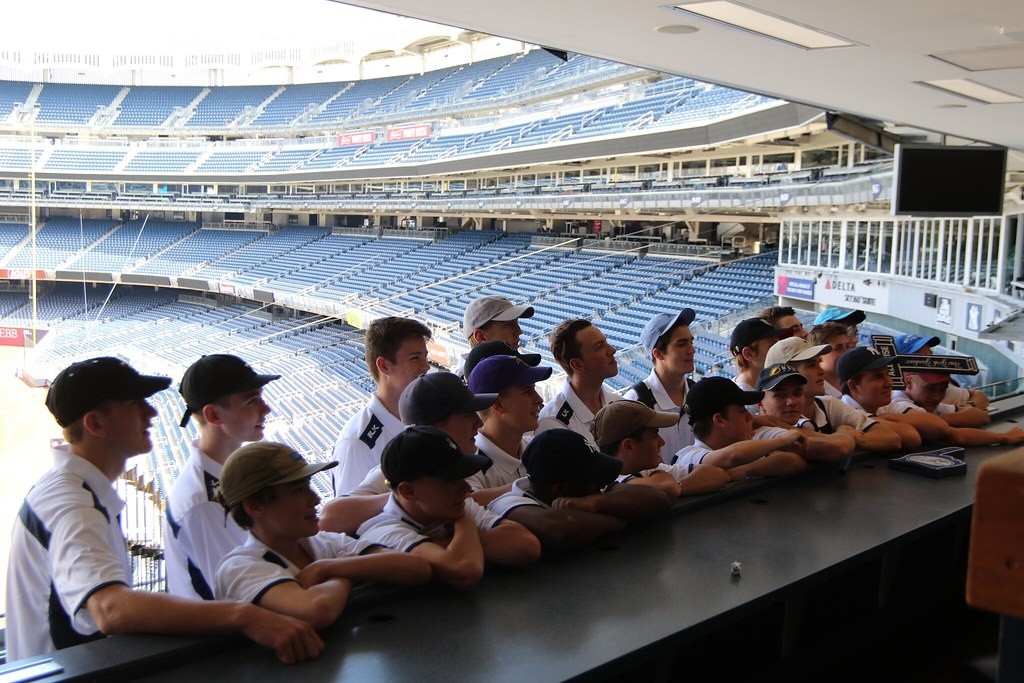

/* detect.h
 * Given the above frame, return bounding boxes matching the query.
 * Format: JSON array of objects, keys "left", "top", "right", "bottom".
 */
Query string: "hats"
[
  {"left": 641, "top": 307, "right": 696, "bottom": 359},
  {"left": 764, "top": 336, "right": 831, "bottom": 368},
  {"left": 686, "top": 376, "right": 765, "bottom": 424},
  {"left": 730, "top": 319, "right": 787, "bottom": 358},
  {"left": 754, "top": 363, "right": 807, "bottom": 392},
  {"left": 45, "top": 356, "right": 173, "bottom": 429},
  {"left": 813, "top": 307, "right": 867, "bottom": 325},
  {"left": 836, "top": 346, "right": 901, "bottom": 384},
  {"left": 464, "top": 343, "right": 541, "bottom": 380},
  {"left": 178, "top": 353, "right": 282, "bottom": 428},
  {"left": 520, "top": 428, "right": 622, "bottom": 489},
  {"left": 217, "top": 441, "right": 339, "bottom": 528},
  {"left": 904, "top": 371, "right": 960, "bottom": 387},
  {"left": 895, "top": 335, "right": 940, "bottom": 354},
  {"left": 380, "top": 426, "right": 489, "bottom": 486},
  {"left": 462, "top": 295, "right": 535, "bottom": 340},
  {"left": 590, "top": 399, "right": 680, "bottom": 447},
  {"left": 398, "top": 373, "right": 499, "bottom": 426}
]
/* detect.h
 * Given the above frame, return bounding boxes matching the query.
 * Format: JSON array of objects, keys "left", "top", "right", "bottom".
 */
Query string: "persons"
[
  {"left": 319, "top": 372, "right": 498, "bottom": 538},
  {"left": 751, "top": 337, "right": 901, "bottom": 468},
  {"left": 6, "top": 355, "right": 322, "bottom": 672},
  {"left": 162, "top": 353, "right": 282, "bottom": 600},
  {"left": 621, "top": 308, "right": 699, "bottom": 464},
  {"left": 357, "top": 425, "right": 542, "bottom": 585},
  {"left": 331, "top": 317, "right": 431, "bottom": 498},
  {"left": 536, "top": 319, "right": 624, "bottom": 452},
  {"left": 887, "top": 333, "right": 1024, "bottom": 447},
  {"left": 670, "top": 376, "right": 807, "bottom": 485},
  {"left": 592, "top": 401, "right": 731, "bottom": 501},
  {"left": 463, "top": 340, "right": 554, "bottom": 503},
  {"left": 214, "top": 440, "right": 429, "bottom": 627},
  {"left": 487, "top": 428, "right": 668, "bottom": 549},
  {"left": 838, "top": 347, "right": 949, "bottom": 447},
  {"left": 726, "top": 307, "right": 807, "bottom": 413},
  {"left": 455, "top": 293, "right": 535, "bottom": 380},
  {"left": 806, "top": 306, "right": 866, "bottom": 400}
]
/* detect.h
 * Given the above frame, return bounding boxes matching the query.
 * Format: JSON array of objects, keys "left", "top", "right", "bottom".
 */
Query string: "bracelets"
[
  {"left": 962, "top": 401, "right": 975, "bottom": 408},
  {"left": 676, "top": 480, "right": 682, "bottom": 496}
]
